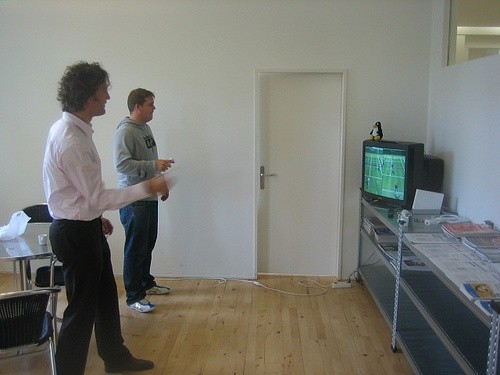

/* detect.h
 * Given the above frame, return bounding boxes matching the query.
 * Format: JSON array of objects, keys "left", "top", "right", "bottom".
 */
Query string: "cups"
[{"left": 38, "top": 233, "right": 47, "bottom": 245}]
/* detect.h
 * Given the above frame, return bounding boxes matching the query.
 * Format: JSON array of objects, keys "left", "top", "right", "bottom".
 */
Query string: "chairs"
[
  {"left": 19, "top": 203, "right": 65, "bottom": 323},
  {"left": 0, "top": 285, "right": 62, "bottom": 375}
]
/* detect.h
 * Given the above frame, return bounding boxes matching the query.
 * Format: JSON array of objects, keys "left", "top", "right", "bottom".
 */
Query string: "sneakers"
[
  {"left": 146, "top": 285, "right": 170, "bottom": 295},
  {"left": 128, "top": 298, "right": 156, "bottom": 313}
]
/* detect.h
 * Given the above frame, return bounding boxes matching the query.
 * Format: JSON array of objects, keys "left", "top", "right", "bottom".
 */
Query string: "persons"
[
  {"left": 43, "top": 62, "right": 176, "bottom": 375},
  {"left": 114, "top": 88, "right": 174, "bottom": 313}
]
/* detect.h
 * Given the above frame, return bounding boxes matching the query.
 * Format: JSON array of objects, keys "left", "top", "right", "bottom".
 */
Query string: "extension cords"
[{"left": 331, "top": 280, "right": 354, "bottom": 289}]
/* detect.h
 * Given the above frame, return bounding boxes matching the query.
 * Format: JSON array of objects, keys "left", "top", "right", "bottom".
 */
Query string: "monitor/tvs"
[{"left": 363, "top": 139, "right": 444, "bottom": 209}]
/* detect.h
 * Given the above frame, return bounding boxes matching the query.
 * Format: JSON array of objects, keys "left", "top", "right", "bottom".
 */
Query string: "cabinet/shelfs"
[{"left": 356, "top": 195, "right": 500, "bottom": 375}]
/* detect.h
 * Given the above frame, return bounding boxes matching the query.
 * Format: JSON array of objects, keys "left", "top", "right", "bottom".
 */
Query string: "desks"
[{"left": 0, "top": 222, "right": 57, "bottom": 290}]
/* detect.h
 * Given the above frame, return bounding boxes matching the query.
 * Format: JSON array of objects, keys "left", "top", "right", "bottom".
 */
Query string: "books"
[
  {"left": 461, "top": 238, "right": 500, "bottom": 251},
  {"left": 363, "top": 216, "right": 386, "bottom": 235},
  {"left": 372, "top": 228, "right": 398, "bottom": 243},
  {"left": 378, "top": 244, "right": 431, "bottom": 271},
  {"left": 460, "top": 284, "right": 500, "bottom": 316},
  {"left": 475, "top": 248, "right": 500, "bottom": 263},
  {"left": 441, "top": 223, "right": 500, "bottom": 239}
]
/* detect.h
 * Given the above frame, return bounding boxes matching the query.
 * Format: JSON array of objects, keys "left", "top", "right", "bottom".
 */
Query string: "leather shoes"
[{"left": 104, "top": 357, "right": 153, "bottom": 373}]
